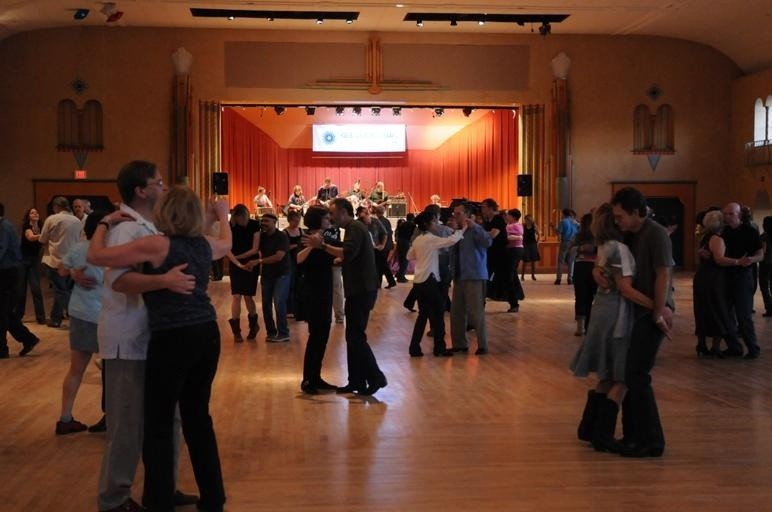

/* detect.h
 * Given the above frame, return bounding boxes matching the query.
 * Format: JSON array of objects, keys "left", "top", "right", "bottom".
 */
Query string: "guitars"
[
  {"left": 377, "top": 192, "right": 404, "bottom": 211},
  {"left": 288, "top": 195, "right": 318, "bottom": 216}
]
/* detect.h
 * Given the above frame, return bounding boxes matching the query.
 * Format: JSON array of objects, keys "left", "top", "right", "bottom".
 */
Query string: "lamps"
[
  {"left": 354, "top": 107, "right": 361, "bottom": 115},
  {"left": 305, "top": 106, "right": 316, "bottom": 115},
  {"left": 450, "top": 21, "right": 458, "bottom": 27},
  {"left": 274, "top": 106, "right": 288, "bottom": 116},
  {"left": 335, "top": 107, "right": 346, "bottom": 115},
  {"left": 266, "top": 16, "right": 275, "bottom": 22},
  {"left": 346, "top": 17, "right": 354, "bottom": 24},
  {"left": 171, "top": 45, "right": 193, "bottom": 184},
  {"left": 416, "top": 20, "right": 423, "bottom": 27},
  {"left": 371, "top": 108, "right": 380, "bottom": 113},
  {"left": 317, "top": 19, "right": 323, "bottom": 24},
  {"left": 551, "top": 52, "right": 571, "bottom": 212},
  {"left": 391, "top": 107, "right": 401, "bottom": 114}
]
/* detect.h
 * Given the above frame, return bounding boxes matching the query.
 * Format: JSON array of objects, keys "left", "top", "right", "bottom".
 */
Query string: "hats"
[{"left": 53, "top": 197, "right": 73, "bottom": 212}]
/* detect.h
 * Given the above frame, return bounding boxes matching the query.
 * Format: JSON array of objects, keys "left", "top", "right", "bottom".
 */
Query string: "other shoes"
[
  {"left": 508, "top": 304, "right": 518, "bottom": 311},
  {"left": 88, "top": 413, "right": 107, "bottom": 432},
  {"left": 359, "top": 381, "right": 387, "bottom": 395},
  {"left": 55, "top": 416, "right": 88, "bottom": 435},
  {"left": 385, "top": 280, "right": 396, "bottom": 288},
  {"left": 266, "top": 332, "right": 275, "bottom": 341},
  {"left": 568, "top": 279, "right": 571, "bottom": 285},
  {"left": 272, "top": 335, "right": 290, "bottom": 342},
  {"left": 301, "top": 381, "right": 318, "bottom": 395},
  {"left": 35, "top": 312, "right": 70, "bottom": 328},
  {"left": 0, "top": 346, "right": 9, "bottom": 358},
  {"left": 621, "top": 440, "right": 663, "bottom": 458},
  {"left": 602, "top": 436, "right": 627, "bottom": 454},
  {"left": 176, "top": 489, "right": 199, "bottom": 506},
  {"left": 316, "top": 380, "right": 337, "bottom": 390},
  {"left": 336, "top": 317, "right": 343, "bottom": 324},
  {"left": 336, "top": 382, "right": 366, "bottom": 392},
  {"left": 694, "top": 326, "right": 758, "bottom": 361},
  {"left": 195, "top": 499, "right": 223, "bottom": 512},
  {"left": 404, "top": 305, "right": 489, "bottom": 358},
  {"left": 397, "top": 277, "right": 408, "bottom": 283},
  {"left": 99, "top": 497, "right": 143, "bottom": 512},
  {"left": 554, "top": 279, "right": 560, "bottom": 285},
  {"left": 19, "top": 333, "right": 40, "bottom": 356},
  {"left": 574, "top": 329, "right": 583, "bottom": 337}
]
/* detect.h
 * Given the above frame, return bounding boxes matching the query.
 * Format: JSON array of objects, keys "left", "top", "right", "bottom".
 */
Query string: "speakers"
[
  {"left": 518, "top": 175, "right": 532, "bottom": 196},
  {"left": 213, "top": 172, "right": 227, "bottom": 194}
]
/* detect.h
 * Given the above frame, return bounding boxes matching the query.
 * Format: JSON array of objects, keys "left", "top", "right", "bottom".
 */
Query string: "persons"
[
  {"left": 2, "top": 163, "right": 232, "bottom": 511},
  {"left": 226, "top": 198, "right": 397, "bottom": 394},
  {"left": 254, "top": 187, "right": 272, "bottom": 208},
  {"left": 288, "top": 186, "right": 305, "bottom": 214},
  {"left": 694, "top": 203, "right": 772, "bottom": 357},
  {"left": 370, "top": 182, "right": 388, "bottom": 204},
  {"left": 554, "top": 187, "right": 678, "bottom": 456},
  {"left": 346, "top": 179, "right": 365, "bottom": 200},
  {"left": 395, "top": 199, "right": 540, "bottom": 356},
  {"left": 322, "top": 177, "right": 338, "bottom": 198}
]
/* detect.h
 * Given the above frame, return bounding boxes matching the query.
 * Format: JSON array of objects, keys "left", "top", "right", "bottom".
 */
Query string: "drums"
[{"left": 257, "top": 206, "right": 275, "bottom": 219}]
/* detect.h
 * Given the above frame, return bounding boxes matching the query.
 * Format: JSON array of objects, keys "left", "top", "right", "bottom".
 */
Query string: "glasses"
[{"left": 146, "top": 179, "right": 164, "bottom": 190}]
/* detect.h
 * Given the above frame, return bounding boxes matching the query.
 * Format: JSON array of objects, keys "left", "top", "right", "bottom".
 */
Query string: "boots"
[
  {"left": 247, "top": 314, "right": 259, "bottom": 339},
  {"left": 229, "top": 318, "right": 242, "bottom": 342},
  {"left": 590, "top": 398, "right": 617, "bottom": 449},
  {"left": 577, "top": 391, "right": 607, "bottom": 440}
]
